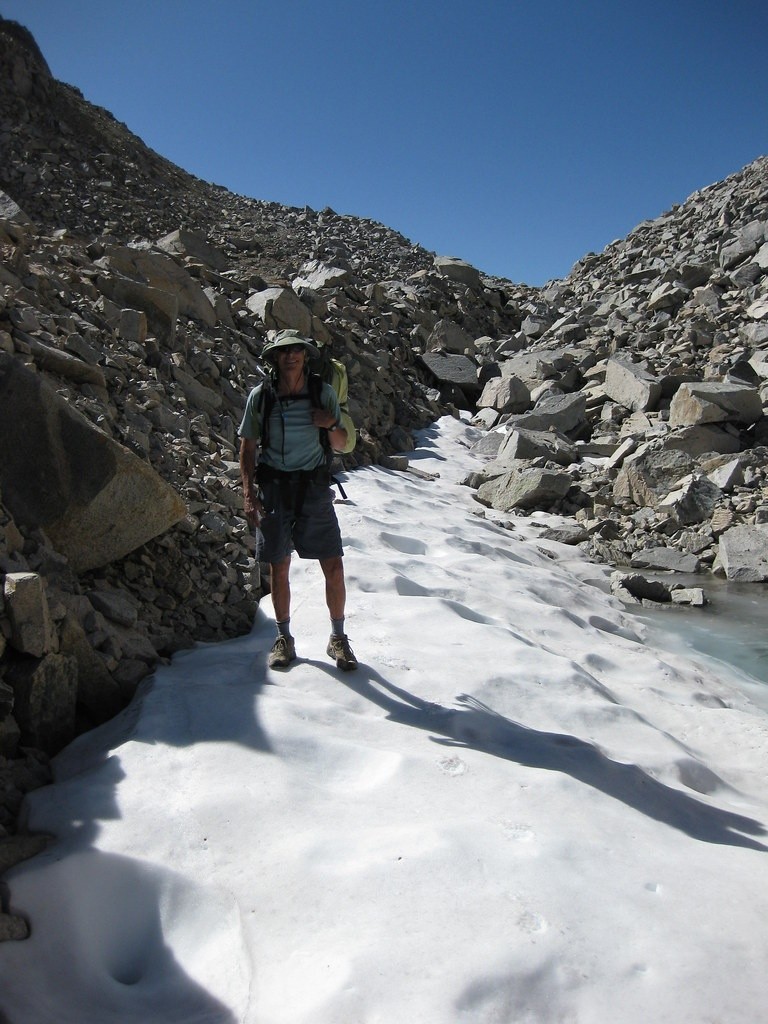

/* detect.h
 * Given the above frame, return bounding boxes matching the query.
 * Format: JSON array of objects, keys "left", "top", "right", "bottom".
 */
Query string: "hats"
[{"left": 262, "top": 328, "right": 320, "bottom": 364}]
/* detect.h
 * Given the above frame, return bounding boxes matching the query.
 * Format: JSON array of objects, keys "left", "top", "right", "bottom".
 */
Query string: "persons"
[{"left": 237, "top": 328, "right": 360, "bottom": 671}]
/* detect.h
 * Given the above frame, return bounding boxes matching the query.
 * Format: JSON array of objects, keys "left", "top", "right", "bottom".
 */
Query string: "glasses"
[{"left": 275, "top": 343, "right": 307, "bottom": 353}]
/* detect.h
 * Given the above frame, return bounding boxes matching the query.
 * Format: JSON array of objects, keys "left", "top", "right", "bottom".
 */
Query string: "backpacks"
[{"left": 258, "top": 358, "right": 357, "bottom": 499}]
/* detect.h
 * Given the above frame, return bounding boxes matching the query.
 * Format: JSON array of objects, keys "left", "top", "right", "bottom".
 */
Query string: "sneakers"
[
  {"left": 327, "top": 634, "right": 358, "bottom": 672},
  {"left": 268, "top": 636, "right": 296, "bottom": 670}
]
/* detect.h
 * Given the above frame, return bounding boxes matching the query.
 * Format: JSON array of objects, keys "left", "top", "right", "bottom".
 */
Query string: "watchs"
[{"left": 327, "top": 422, "right": 337, "bottom": 431}]
